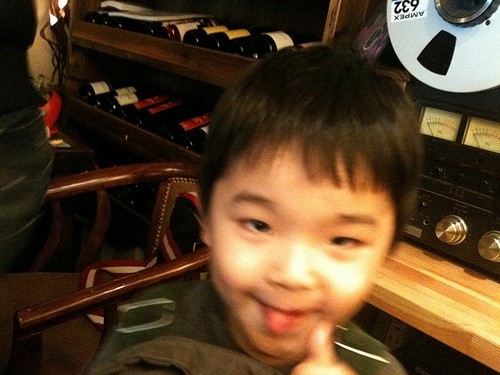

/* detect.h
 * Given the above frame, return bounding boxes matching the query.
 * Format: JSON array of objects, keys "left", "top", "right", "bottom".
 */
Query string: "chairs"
[{"left": 0, "top": 163, "right": 218, "bottom": 375}]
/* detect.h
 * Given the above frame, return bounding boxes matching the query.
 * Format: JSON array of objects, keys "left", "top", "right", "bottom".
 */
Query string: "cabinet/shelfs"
[{"left": 51, "top": 0, "right": 379, "bottom": 207}]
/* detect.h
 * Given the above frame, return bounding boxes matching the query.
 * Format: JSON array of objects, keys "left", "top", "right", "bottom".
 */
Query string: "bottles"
[
  {"left": 76, "top": 79, "right": 214, "bottom": 155},
  {"left": 352, "top": 0, "right": 389, "bottom": 63},
  {"left": 84, "top": 8, "right": 316, "bottom": 61}
]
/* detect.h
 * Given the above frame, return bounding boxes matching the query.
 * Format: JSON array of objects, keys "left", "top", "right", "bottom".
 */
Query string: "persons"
[{"left": 83, "top": 41, "right": 424, "bottom": 375}]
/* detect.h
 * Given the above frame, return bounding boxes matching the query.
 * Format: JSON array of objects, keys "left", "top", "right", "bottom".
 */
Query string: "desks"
[{"left": 351, "top": 240, "right": 500, "bottom": 375}]
